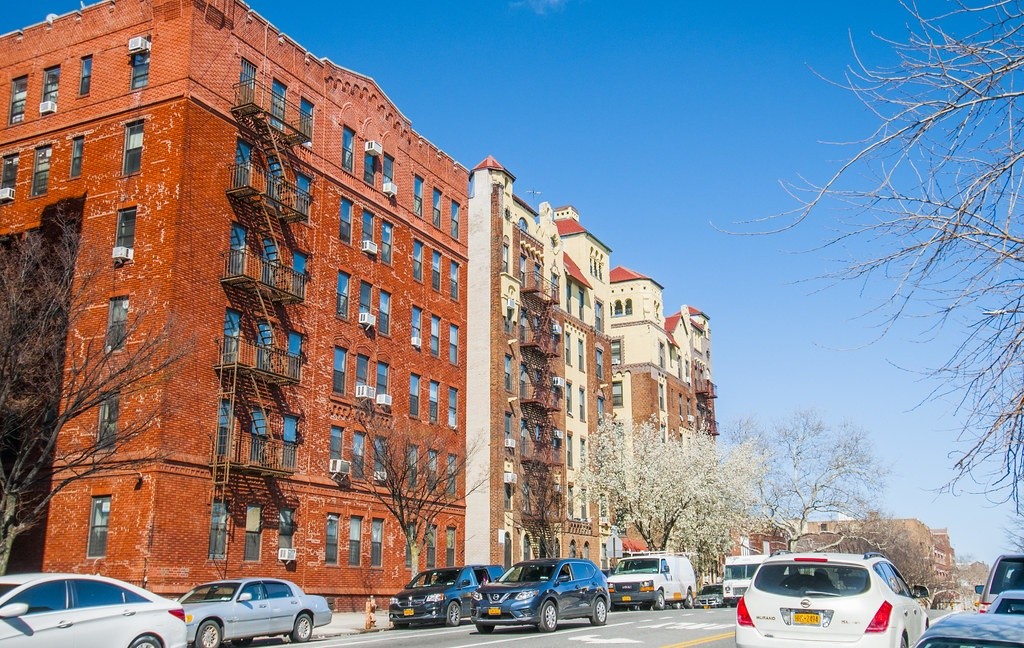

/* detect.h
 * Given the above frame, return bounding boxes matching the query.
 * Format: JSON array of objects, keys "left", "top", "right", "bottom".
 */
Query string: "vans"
[
  {"left": 388, "top": 564, "right": 509, "bottom": 629},
  {"left": 607, "top": 550, "right": 699, "bottom": 611}
]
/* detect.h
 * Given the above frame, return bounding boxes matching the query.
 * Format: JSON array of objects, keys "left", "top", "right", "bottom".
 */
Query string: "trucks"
[{"left": 722, "top": 555, "right": 771, "bottom": 608}]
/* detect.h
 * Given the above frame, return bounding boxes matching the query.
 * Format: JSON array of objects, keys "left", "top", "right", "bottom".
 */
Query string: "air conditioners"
[
  {"left": 612, "top": 358, "right": 618, "bottom": 364},
  {"left": 505, "top": 430, "right": 563, "bottom": 492},
  {"left": 40, "top": 101, "right": 57, "bottom": 113},
  {"left": 361, "top": 141, "right": 397, "bottom": 254},
  {"left": 552, "top": 377, "right": 564, "bottom": 387},
  {"left": 127, "top": 36, "right": 149, "bottom": 50},
  {"left": 278, "top": 548, "right": 296, "bottom": 560},
  {"left": 329, "top": 313, "right": 422, "bottom": 480},
  {"left": 553, "top": 325, "right": 563, "bottom": 334},
  {"left": 111, "top": 246, "right": 133, "bottom": 259},
  {"left": 687, "top": 415, "right": 694, "bottom": 422},
  {"left": 0, "top": 188, "right": 16, "bottom": 200},
  {"left": 686, "top": 377, "right": 690, "bottom": 383}
]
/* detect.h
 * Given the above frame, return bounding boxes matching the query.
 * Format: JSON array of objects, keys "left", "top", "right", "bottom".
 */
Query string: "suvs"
[{"left": 736, "top": 550, "right": 930, "bottom": 648}]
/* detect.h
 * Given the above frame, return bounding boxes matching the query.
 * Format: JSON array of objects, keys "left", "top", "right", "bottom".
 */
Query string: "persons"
[{"left": 813, "top": 569, "right": 835, "bottom": 591}]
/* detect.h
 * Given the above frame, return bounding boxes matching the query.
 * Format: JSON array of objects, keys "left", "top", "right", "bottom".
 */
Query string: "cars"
[
  {"left": 470, "top": 558, "right": 611, "bottom": 635},
  {"left": 1, "top": 572, "right": 189, "bottom": 648},
  {"left": 913, "top": 612, "right": 1024, "bottom": 647},
  {"left": 985, "top": 590, "right": 1024, "bottom": 615},
  {"left": 178, "top": 577, "right": 333, "bottom": 648},
  {"left": 694, "top": 584, "right": 727, "bottom": 608},
  {"left": 975, "top": 555, "right": 1024, "bottom": 613}
]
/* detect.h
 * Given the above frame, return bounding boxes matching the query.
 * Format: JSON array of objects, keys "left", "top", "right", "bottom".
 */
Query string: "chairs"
[
  {"left": 1004, "top": 571, "right": 1024, "bottom": 591},
  {"left": 843, "top": 576, "right": 863, "bottom": 596}
]
